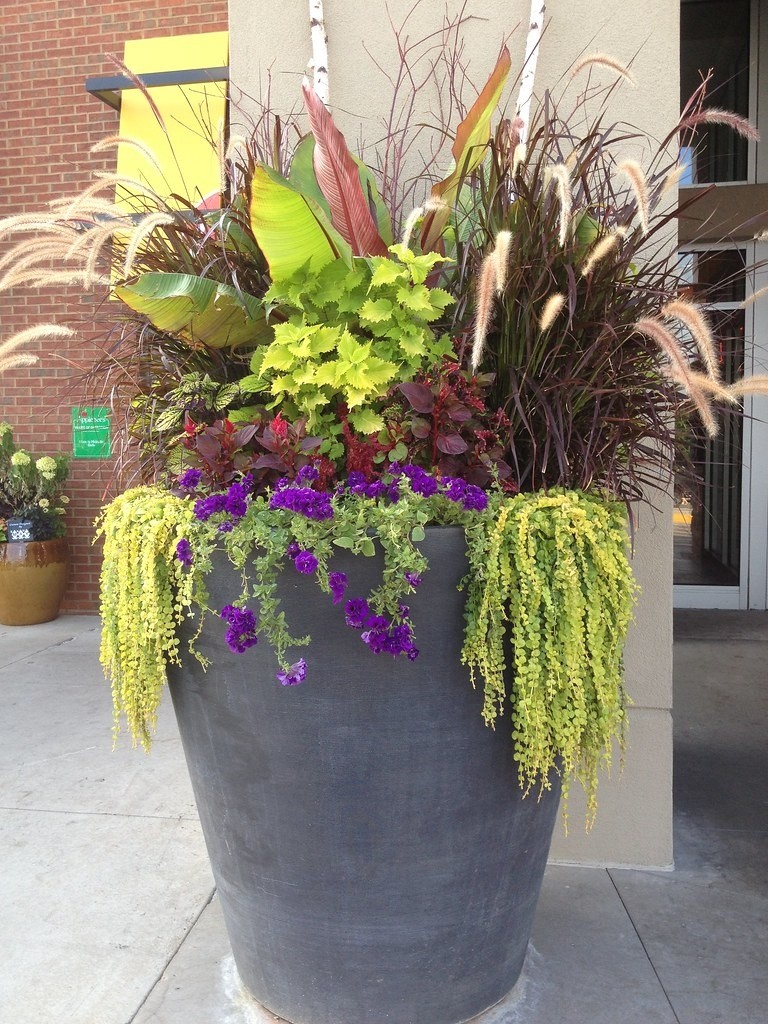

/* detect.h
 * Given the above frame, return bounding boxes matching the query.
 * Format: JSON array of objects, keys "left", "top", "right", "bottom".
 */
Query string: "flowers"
[
  {"left": 0, "top": 42, "right": 768, "bottom": 833},
  {"left": 0, "top": 420, "right": 73, "bottom": 544}
]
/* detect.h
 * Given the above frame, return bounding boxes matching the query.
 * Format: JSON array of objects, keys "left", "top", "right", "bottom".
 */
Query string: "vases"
[
  {"left": 148, "top": 520, "right": 586, "bottom": 1024},
  {"left": 0, "top": 538, "right": 71, "bottom": 626}
]
